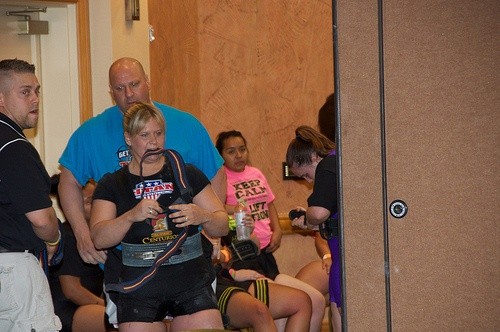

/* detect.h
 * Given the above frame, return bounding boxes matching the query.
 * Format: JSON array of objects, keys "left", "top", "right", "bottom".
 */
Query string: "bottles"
[{"left": 234, "top": 199, "right": 251, "bottom": 240}]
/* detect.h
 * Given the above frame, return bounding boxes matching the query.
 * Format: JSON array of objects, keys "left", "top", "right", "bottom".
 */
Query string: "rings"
[
  {"left": 185, "top": 216, "right": 189, "bottom": 221},
  {"left": 149, "top": 208, "right": 152, "bottom": 214}
]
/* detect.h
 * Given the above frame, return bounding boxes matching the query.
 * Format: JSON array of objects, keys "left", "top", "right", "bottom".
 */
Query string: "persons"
[
  {"left": 58, "top": 57, "right": 227, "bottom": 332},
  {"left": 0, "top": 58, "right": 62, "bottom": 332},
  {"left": 212, "top": 93, "right": 342, "bottom": 332},
  {"left": 48, "top": 174, "right": 105, "bottom": 332},
  {"left": 89, "top": 103, "right": 230, "bottom": 332}
]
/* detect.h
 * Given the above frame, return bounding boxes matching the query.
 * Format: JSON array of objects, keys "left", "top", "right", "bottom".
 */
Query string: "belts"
[{"left": 0, "top": 244, "right": 35, "bottom": 253}]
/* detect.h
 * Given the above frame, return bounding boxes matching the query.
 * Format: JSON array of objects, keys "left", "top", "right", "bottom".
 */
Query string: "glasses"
[{"left": 110, "top": 77, "right": 144, "bottom": 93}]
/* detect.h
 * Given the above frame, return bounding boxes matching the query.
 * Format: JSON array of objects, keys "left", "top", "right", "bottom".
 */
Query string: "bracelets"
[
  {"left": 44, "top": 231, "right": 61, "bottom": 245},
  {"left": 322, "top": 254, "right": 331, "bottom": 261},
  {"left": 230, "top": 268, "right": 235, "bottom": 279}
]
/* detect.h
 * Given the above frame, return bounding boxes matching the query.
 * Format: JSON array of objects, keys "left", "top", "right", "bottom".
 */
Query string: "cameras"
[{"left": 289, "top": 209, "right": 338, "bottom": 234}]
